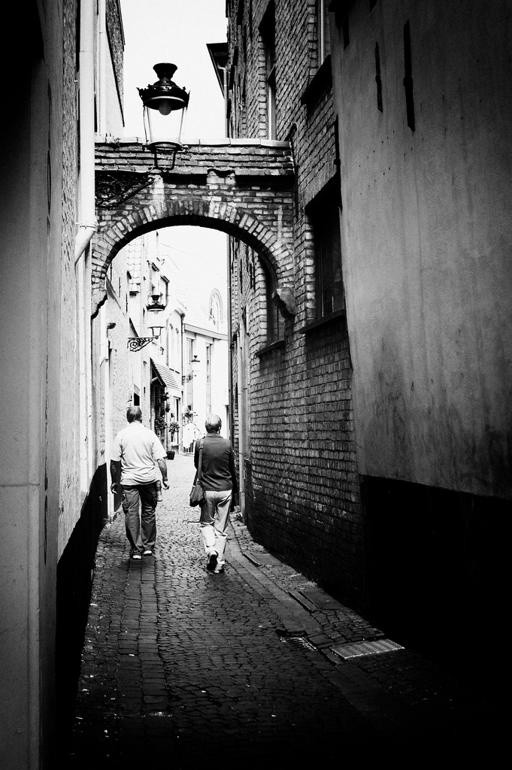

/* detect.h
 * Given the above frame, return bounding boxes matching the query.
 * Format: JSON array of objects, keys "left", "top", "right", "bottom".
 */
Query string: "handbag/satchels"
[{"left": 190, "top": 485, "right": 205, "bottom": 507}]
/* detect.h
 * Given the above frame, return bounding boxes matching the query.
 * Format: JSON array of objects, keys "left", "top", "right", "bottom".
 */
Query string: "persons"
[
  {"left": 193, "top": 416, "right": 240, "bottom": 575},
  {"left": 110, "top": 406, "right": 169, "bottom": 559}
]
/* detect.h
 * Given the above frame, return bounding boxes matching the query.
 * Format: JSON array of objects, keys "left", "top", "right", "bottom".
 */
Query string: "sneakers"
[
  {"left": 133, "top": 550, "right": 152, "bottom": 560},
  {"left": 207, "top": 552, "right": 225, "bottom": 574}
]
